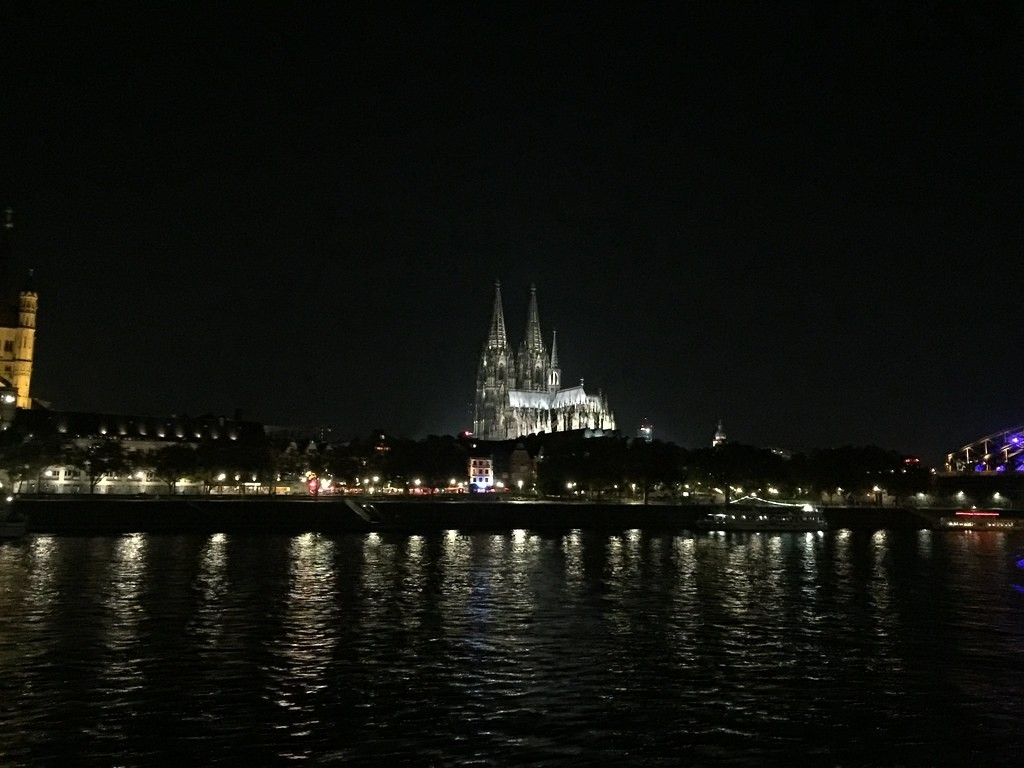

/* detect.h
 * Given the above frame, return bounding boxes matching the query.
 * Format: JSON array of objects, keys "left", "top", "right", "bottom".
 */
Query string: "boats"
[
  {"left": 935, "top": 517, "right": 1024, "bottom": 530},
  {"left": 695, "top": 509, "right": 829, "bottom": 532}
]
